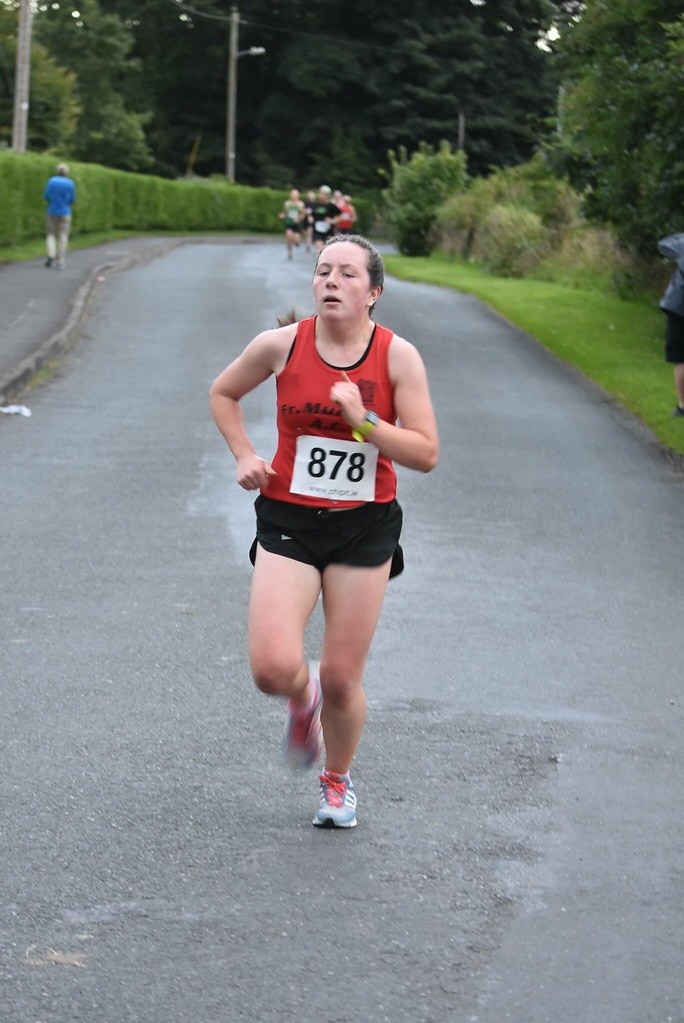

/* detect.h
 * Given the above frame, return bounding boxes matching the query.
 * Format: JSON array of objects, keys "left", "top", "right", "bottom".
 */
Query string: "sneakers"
[
  {"left": 316, "top": 771, "right": 357, "bottom": 829},
  {"left": 280, "top": 665, "right": 328, "bottom": 767}
]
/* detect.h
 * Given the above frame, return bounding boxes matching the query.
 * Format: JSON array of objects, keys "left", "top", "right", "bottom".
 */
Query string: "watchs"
[{"left": 353, "top": 407, "right": 380, "bottom": 445}]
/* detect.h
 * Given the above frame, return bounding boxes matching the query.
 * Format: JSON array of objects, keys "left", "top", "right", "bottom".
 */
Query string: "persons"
[
  {"left": 651, "top": 229, "right": 684, "bottom": 415},
  {"left": 211, "top": 233, "right": 445, "bottom": 830},
  {"left": 44, "top": 164, "right": 74, "bottom": 270},
  {"left": 278, "top": 186, "right": 359, "bottom": 259}
]
